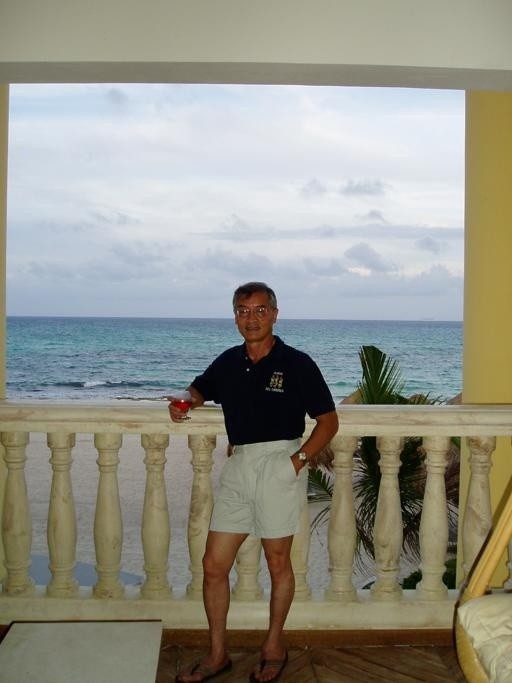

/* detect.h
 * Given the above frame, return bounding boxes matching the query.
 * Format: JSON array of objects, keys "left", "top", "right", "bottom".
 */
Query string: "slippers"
[
  {"left": 249, "top": 646, "right": 289, "bottom": 683},
  {"left": 177, "top": 658, "right": 233, "bottom": 681}
]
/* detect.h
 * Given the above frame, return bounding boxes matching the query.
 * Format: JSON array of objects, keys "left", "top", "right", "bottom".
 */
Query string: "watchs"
[{"left": 295, "top": 449, "right": 309, "bottom": 467}]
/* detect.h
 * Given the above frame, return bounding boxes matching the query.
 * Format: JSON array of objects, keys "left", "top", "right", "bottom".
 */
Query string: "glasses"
[{"left": 235, "top": 306, "right": 267, "bottom": 315}]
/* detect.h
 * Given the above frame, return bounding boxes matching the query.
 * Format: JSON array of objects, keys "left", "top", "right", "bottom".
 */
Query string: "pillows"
[{"left": 456, "top": 587, "right": 512, "bottom": 683}]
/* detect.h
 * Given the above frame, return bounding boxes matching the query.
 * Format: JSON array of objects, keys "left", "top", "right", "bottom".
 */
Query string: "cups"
[{"left": 173, "top": 390, "right": 192, "bottom": 419}]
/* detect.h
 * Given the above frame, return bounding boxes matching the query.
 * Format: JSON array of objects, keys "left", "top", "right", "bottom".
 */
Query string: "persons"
[{"left": 166, "top": 282, "right": 339, "bottom": 682}]
